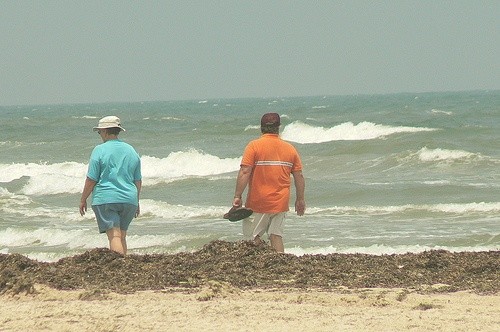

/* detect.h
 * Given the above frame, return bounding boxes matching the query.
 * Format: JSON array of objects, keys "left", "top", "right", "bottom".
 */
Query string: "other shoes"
[{"left": 224, "top": 204, "right": 253, "bottom": 222}]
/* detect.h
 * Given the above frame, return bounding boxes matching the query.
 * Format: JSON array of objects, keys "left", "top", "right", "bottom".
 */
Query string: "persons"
[
  {"left": 233, "top": 114, "right": 305, "bottom": 252},
  {"left": 80, "top": 116, "right": 142, "bottom": 255}
]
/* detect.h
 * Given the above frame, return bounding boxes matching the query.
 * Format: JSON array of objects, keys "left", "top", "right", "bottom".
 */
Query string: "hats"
[
  {"left": 92, "top": 115, "right": 126, "bottom": 132},
  {"left": 261, "top": 112, "right": 281, "bottom": 127}
]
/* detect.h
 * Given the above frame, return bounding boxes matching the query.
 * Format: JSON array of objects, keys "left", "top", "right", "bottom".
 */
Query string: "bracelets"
[{"left": 233, "top": 196, "right": 241, "bottom": 199}]
